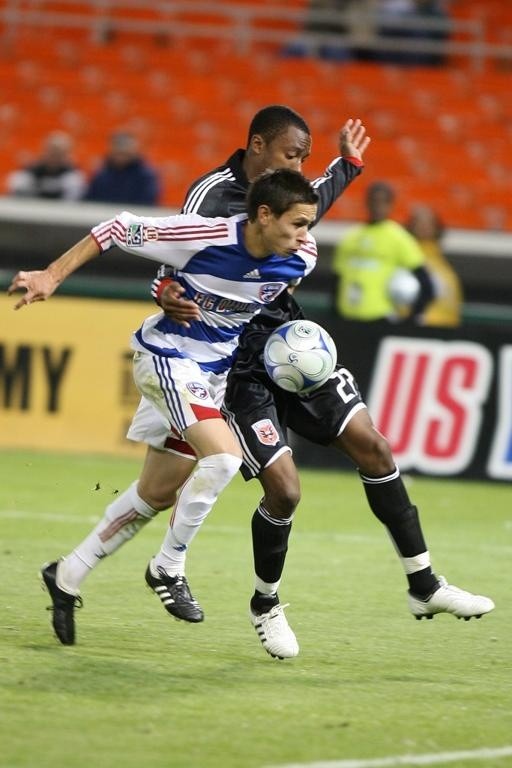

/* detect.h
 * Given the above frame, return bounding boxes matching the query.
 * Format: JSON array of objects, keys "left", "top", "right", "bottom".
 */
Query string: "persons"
[
  {"left": 6, "top": 130, "right": 85, "bottom": 199},
  {"left": 329, "top": 180, "right": 433, "bottom": 324},
  {"left": 8, "top": 166, "right": 319, "bottom": 645},
  {"left": 396, "top": 206, "right": 462, "bottom": 327},
  {"left": 86, "top": 133, "right": 161, "bottom": 204},
  {"left": 151, "top": 103, "right": 494, "bottom": 660}
]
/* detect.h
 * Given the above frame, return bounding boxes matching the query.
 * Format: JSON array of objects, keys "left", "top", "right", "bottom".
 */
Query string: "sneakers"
[
  {"left": 408, "top": 584, "right": 495, "bottom": 620},
  {"left": 41, "top": 560, "right": 77, "bottom": 646},
  {"left": 145, "top": 560, "right": 203, "bottom": 623},
  {"left": 249, "top": 595, "right": 299, "bottom": 659}
]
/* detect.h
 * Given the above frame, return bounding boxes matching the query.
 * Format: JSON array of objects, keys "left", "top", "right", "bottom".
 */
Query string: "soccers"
[
  {"left": 387, "top": 269, "right": 421, "bottom": 307},
  {"left": 264, "top": 319, "right": 338, "bottom": 395}
]
